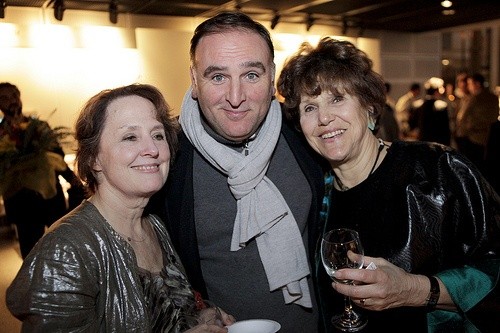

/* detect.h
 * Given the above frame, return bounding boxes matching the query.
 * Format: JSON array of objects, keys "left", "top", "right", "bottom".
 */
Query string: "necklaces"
[
  {"left": 112, "top": 224, "right": 150, "bottom": 242},
  {"left": 328, "top": 137, "right": 388, "bottom": 192}
]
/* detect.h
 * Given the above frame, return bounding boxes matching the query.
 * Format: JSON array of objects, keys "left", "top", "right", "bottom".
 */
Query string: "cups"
[{"left": 224, "top": 319, "right": 282, "bottom": 333}]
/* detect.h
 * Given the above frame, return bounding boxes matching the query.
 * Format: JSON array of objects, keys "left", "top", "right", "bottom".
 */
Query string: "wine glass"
[{"left": 320, "top": 229, "right": 368, "bottom": 332}]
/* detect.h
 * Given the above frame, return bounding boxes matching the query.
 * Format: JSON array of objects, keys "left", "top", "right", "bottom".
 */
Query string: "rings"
[{"left": 360, "top": 297, "right": 367, "bottom": 306}]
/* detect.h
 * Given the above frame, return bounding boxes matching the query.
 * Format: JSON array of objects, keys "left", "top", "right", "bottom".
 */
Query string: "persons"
[
  {"left": 274, "top": 35, "right": 500, "bottom": 333},
  {"left": 383, "top": 70, "right": 499, "bottom": 162},
  {"left": 143, "top": 8, "right": 332, "bottom": 333},
  {"left": 0, "top": 81, "right": 85, "bottom": 261},
  {"left": 4, "top": 81, "right": 240, "bottom": 333}
]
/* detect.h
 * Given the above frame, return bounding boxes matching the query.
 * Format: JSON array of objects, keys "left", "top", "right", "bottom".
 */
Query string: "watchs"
[{"left": 422, "top": 272, "right": 441, "bottom": 309}]
